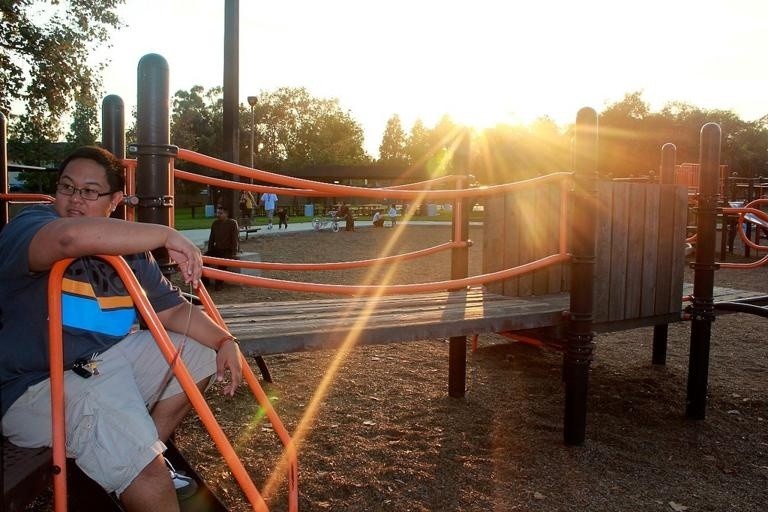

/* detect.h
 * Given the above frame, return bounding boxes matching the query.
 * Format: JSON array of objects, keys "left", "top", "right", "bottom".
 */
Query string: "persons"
[
  {"left": 373, "top": 209, "right": 384, "bottom": 227},
  {"left": 257, "top": 193, "right": 279, "bottom": 229},
  {"left": 388, "top": 203, "right": 397, "bottom": 226},
  {"left": 240, "top": 189, "right": 258, "bottom": 228},
  {"left": 277, "top": 207, "right": 288, "bottom": 228},
  {"left": 333, "top": 202, "right": 348, "bottom": 224},
  {"left": 201, "top": 207, "right": 240, "bottom": 291},
  {"left": 0, "top": 144, "right": 243, "bottom": 512}
]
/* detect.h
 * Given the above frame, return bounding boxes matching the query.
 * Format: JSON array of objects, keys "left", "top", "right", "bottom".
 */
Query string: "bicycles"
[{"left": 312, "top": 210, "right": 340, "bottom": 232}]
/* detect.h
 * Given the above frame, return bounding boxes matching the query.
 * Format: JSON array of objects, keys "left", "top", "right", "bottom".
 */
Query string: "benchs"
[
  {"left": 2, "top": 435, "right": 53, "bottom": 510},
  {"left": 180, "top": 285, "right": 571, "bottom": 401},
  {"left": 227, "top": 251, "right": 260, "bottom": 290},
  {"left": 239, "top": 218, "right": 261, "bottom": 240}
]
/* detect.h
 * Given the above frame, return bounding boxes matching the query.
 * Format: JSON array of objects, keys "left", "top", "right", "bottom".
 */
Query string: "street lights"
[{"left": 247, "top": 97, "right": 258, "bottom": 194}]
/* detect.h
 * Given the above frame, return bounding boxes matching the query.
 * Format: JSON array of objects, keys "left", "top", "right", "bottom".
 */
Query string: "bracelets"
[{"left": 217, "top": 336, "right": 239, "bottom": 349}]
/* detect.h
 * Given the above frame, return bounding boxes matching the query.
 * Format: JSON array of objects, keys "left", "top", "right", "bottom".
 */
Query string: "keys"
[{"left": 71, "top": 357, "right": 103, "bottom": 378}]
